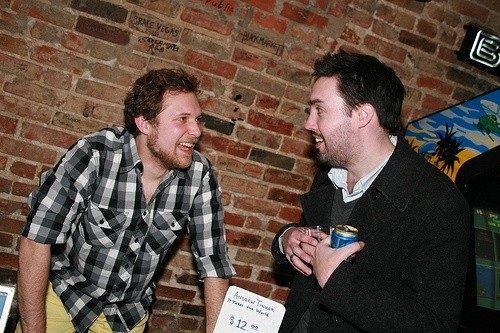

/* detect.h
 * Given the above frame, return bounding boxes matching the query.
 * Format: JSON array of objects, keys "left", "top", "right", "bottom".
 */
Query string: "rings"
[{"left": 289, "top": 253, "right": 296, "bottom": 263}]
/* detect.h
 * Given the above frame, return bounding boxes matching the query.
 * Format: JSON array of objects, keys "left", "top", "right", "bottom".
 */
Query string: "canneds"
[{"left": 330, "top": 225, "right": 358, "bottom": 264}]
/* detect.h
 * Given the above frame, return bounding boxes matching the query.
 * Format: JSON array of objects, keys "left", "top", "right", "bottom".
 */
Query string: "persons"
[
  {"left": 14, "top": 68, "right": 238, "bottom": 333},
  {"left": 269, "top": 49, "right": 481, "bottom": 333}
]
